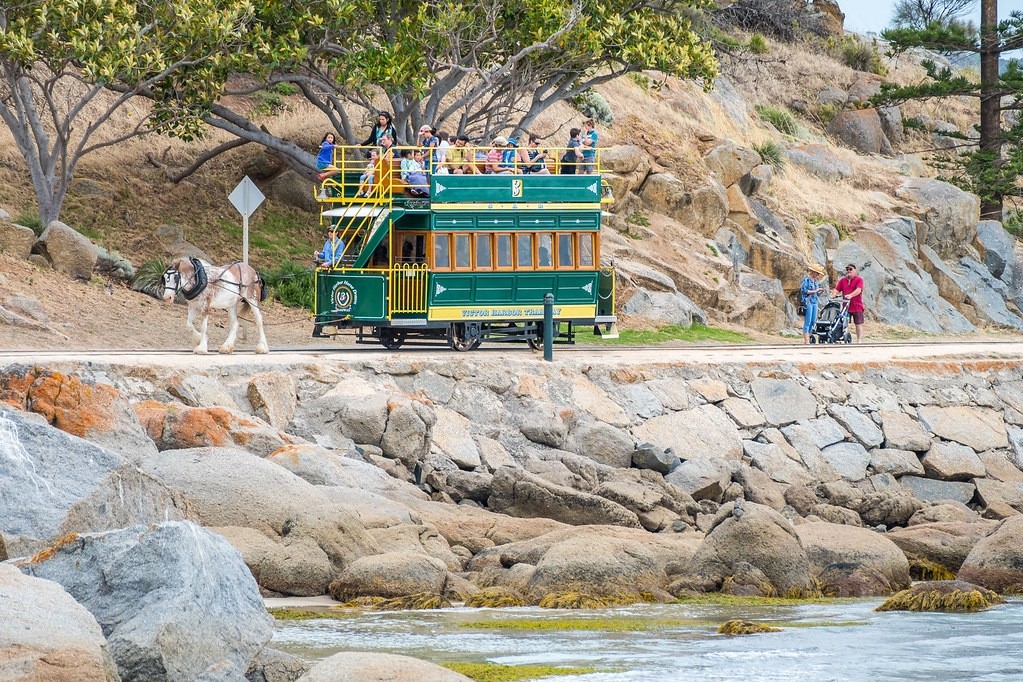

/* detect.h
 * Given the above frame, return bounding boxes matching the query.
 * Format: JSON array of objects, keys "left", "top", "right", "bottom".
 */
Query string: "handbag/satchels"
[
  {"left": 531, "top": 152, "right": 543, "bottom": 172},
  {"left": 797, "top": 305, "right": 807, "bottom": 316}
]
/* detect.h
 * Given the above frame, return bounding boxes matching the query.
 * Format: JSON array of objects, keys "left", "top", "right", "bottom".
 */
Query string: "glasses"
[
  {"left": 379, "top": 112, "right": 388, "bottom": 117},
  {"left": 420, "top": 130, "right": 429, "bottom": 133},
  {"left": 328, "top": 230, "right": 337, "bottom": 233},
  {"left": 846, "top": 267, "right": 853, "bottom": 271},
  {"left": 532, "top": 139, "right": 540, "bottom": 145}
]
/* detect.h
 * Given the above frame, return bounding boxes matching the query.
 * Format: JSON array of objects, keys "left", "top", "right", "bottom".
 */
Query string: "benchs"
[{"left": 359, "top": 157, "right": 561, "bottom": 194}]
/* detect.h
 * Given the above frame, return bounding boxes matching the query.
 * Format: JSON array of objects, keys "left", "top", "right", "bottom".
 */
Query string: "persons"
[
  {"left": 402, "top": 239, "right": 414, "bottom": 266},
  {"left": 418, "top": 125, "right": 483, "bottom": 174},
  {"left": 830, "top": 264, "right": 866, "bottom": 343},
  {"left": 314, "top": 132, "right": 339, "bottom": 181},
  {"left": 799, "top": 265, "right": 825, "bottom": 345},
  {"left": 478, "top": 133, "right": 524, "bottom": 174},
  {"left": 520, "top": 133, "right": 551, "bottom": 174},
  {"left": 373, "top": 244, "right": 389, "bottom": 266},
  {"left": 560, "top": 127, "right": 591, "bottom": 175},
  {"left": 382, "top": 132, "right": 401, "bottom": 159},
  {"left": 434, "top": 241, "right": 551, "bottom": 266},
  {"left": 400, "top": 149, "right": 430, "bottom": 195},
  {"left": 354, "top": 111, "right": 400, "bottom": 159},
  {"left": 579, "top": 119, "right": 598, "bottom": 174},
  {"left": 357, "top": 148, "right": 381, "bottom": 197},
  {"left": 312, "top": 224, "right": 346, "bottom": 270}
]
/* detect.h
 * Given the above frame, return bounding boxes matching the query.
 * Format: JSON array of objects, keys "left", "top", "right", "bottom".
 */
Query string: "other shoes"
[
  {"left": 420, "top": 190, "right": 423, "bottom": 194},
  {"left": 358, "top": 190, "right": 364, "bottom": 195},
  {"left": 411, "top": 189, "right": 418, "bottom": 195},
  {"left": 316, "top": 173, "right": 324, "bottom": 181},
  {"left": 365, "top": 191, "right": 371, "bottom": 197}
]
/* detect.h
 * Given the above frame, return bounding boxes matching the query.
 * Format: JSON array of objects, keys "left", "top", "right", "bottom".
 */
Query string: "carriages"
[{"left": 160, "top": 144, "right": 618, "bottom": 355}]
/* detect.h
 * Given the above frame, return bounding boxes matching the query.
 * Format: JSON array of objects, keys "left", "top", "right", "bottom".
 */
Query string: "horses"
[{"left": 161, "top": 256, "right": 269, "bottom": 354}]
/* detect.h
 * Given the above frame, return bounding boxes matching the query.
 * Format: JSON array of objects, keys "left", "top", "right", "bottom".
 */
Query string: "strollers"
[{"left": 809, "top": 295, "right": 852, "bottom": 344}]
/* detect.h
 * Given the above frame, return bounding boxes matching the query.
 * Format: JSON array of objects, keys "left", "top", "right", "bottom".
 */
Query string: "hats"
[
  {"left": 507, "top": 138, "right": 519, "bottom": 147},
  {"left": 809, "top": 264, "right": 824, "bottom": 275},
  {"left": 327, "top": 224, "right": 339, "bottom": 232},
  {"left": 846, "top": 265, "right": 856, "bottom": 272},
  {"left": 418, "top": 125, "right": 432, "bottom": 135},
  {"left": 494, "top": 136, "right": 509, "bottom": 146}
]
